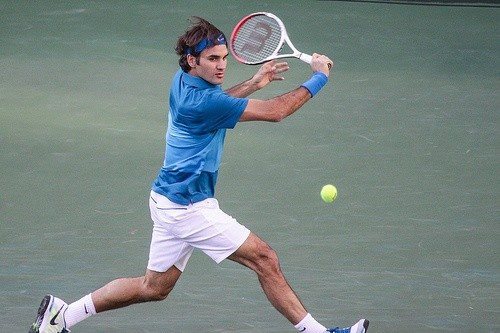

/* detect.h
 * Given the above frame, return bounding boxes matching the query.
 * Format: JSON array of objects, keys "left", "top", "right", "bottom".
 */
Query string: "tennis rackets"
[{"left": 229, "top": 11, "right": 333, "bottom": 72}]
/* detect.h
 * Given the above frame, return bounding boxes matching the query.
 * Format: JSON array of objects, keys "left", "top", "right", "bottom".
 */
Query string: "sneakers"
[
  {"left": 27, "top": 294, "right": 68, "bottom": 333},
  {"left": 324, "top": 318, "right": 371, "bottom": 333}
]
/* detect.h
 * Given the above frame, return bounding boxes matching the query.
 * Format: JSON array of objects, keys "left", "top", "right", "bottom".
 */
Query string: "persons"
[{"left": 28, "top": 14, "right": 371, "bottom": 333}]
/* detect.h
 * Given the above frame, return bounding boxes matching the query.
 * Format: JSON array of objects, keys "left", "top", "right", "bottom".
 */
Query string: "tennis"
[{"left": 320, "top": 185, "right": 337, "bottom": 203}]
